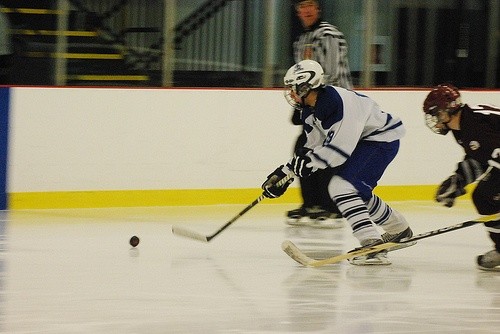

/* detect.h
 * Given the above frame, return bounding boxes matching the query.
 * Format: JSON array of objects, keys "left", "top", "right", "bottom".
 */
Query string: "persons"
[
  {"left": 287, "top": 0, "right": 354, "bottom": 220},
  {"left": 261, "top": 59, "right": 413, "bottom": 265},
  {"left": 422, "top": 85, "right": 500, "bottom": 271}
]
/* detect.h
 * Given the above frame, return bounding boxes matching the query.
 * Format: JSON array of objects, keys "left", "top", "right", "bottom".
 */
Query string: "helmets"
[
  {"left": 283, "top": 60, "right": 325, "bottom": 110},
  {"left": 423, "top": 84, "right": 463, "bottom": 135}
]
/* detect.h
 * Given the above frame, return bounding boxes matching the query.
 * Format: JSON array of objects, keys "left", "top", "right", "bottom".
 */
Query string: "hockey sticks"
[
  {"left": 281, "top": 211, "right": 500, "bottom": 268},
  {"left": 172, "top": 169, "right": 297, "bottom": 243}
]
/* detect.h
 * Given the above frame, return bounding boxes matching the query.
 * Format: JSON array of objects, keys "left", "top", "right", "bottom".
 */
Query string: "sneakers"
[
  {"left": 287, "top": 204, "right": 347, "bottom": 226},
  {"left": 347, "top": 247, "right": 391, "bottom": 265},
  {"left": 476, "top": 249, "right": 500, "bottom": 270},
  {"left": 382, "top": 228, "right": 415, "bottom": 249}
]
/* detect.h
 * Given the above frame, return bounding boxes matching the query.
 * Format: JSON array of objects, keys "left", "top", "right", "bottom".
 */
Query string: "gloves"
[
  {"left": 262, "top": 165, "right": 295, "bottom": 199},
  {"left": 435, "top": 174, "right": 466, "bottom": 208}
]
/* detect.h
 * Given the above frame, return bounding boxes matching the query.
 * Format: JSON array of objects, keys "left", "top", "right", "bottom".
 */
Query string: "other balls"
[{"left": 129, "top": 236, "right": 140, "bottom": 247}]
